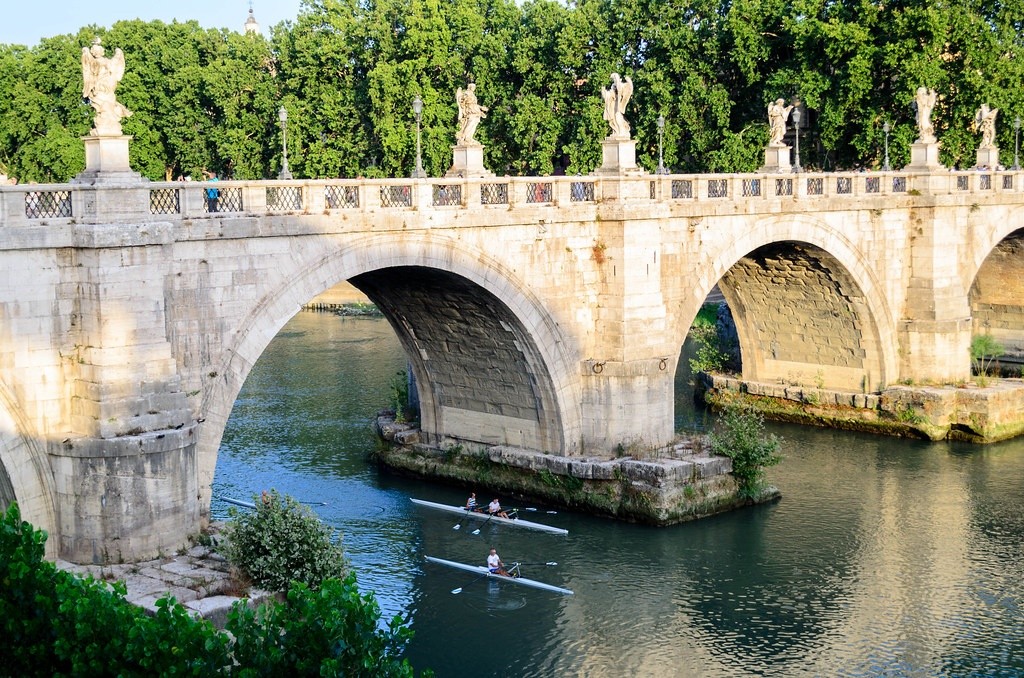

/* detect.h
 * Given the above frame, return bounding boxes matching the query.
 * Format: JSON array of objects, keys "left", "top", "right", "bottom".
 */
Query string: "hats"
[{"left": 184, "top": 176, "right": 191, "bottom": 181}]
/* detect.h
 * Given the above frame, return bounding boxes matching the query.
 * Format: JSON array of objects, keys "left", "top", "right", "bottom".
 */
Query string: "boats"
[
  {"left": 409, "top": 498, "right": 569, "bottom": 535},
  {"left": 423, "top": 555, "right": 574, "bottom": 595}
]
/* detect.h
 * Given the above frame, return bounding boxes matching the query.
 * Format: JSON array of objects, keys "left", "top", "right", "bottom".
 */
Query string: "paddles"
[
  {"left": 282, "top": 500, "right": 328, "bottom": 505},
  {"left": 450, "top": 571, "right": 494, "bottom": 595},
  {"left": 500, "top": 505, "right": 559, "bottom": 515},
  {"left": 452, "top": 508, "right": 472, "bottom": 531},
  {"left": 501, "top": 561, "right": 559, "bottom": 567},
  {"left": 471, "top": 514, "right": 494, "bottom": 535}
]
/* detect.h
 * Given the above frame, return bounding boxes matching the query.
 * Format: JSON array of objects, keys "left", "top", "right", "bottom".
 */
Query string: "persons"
[
  {"left": 535, "top": 173, "right": 550, "bottom": 202},
  {"left": 574, "top": 172, "right": 594, "bottom": 201},
  {"left": 480, "top": 173, "right": 511, "bottom": 200},
  {"left": 260, "top": 491, "right": 272, "bottom": 508},
  {"left": 950, "top": 161, "right": 1006, "bottom": 189},
  {"left": 12, "top": 177, "right": 41, "bottom": 218},
  {"left": 462, "top": 83, "right": 488, "bottom": 142},
  {"left": 980, "top": 103, "right": 994, "bottom": 147},
  {"left": 466, "top": 493, "right": 483, "bottom": 513},
  {"left": 382, "top": 177, "right": 391, "bottom": 204},
  {"left": 916, "top": 86, "right": 931, "bottom": 139},
  {"left": 203, "top": 171, "right": 219, "bottom": 213},
  {"left": 807, "top": 166, "right": 900, "bottom": 194},
  {"left": 326, "top": 173, "right": 366, "bottom": 208},
  {"left": 770, "top": 99, "right": 786, "bottom": 144},
  {"left": 438, "top": 174, "right": 463, "bottom": 205},
  {"left": 737, "top": 170, "right": 758, "bottom": 195},
  {"left": 175, "top": 175, "right": 191, "bottom": 202},
  {"left": 489, "top": 497, "right": 509, "bottom": 519},
  {"left": 487, "top": 548, "right": 511, "bottom": 577},
  {"left": 91, "top": 45, "right": 133, "bottom": 128},
  {"left": 600, "top": 72, "right": 623, "bottom": 134}
]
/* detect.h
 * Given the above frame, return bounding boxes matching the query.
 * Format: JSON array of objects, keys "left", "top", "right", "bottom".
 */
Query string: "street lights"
[
  {"left": 792, "top": 106, "right": 803, "bottom": 172},
  {"left": 413, "top": 96, "right": 427, "bottom": 178},
  {"left": 278, "top": 105, "right": 293, "bottom": 180},
  {"left": 883, "top": 121, "right": 891, "bottom": 171},
  {"left": 1012, "top": 116, "right": 1021, "bottom": 170},
  {"left": 656, "top": 113, "right": 667, "bottom": 174}
]
